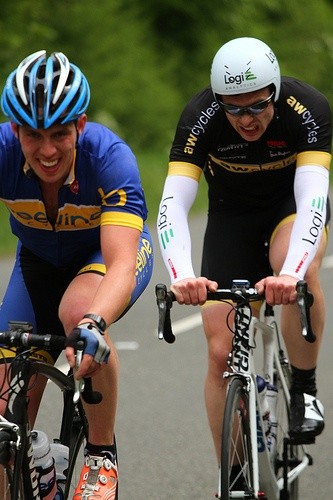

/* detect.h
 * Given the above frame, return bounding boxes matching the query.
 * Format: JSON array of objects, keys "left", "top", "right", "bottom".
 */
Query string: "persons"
[
  {"left": 154, "top": 35, "right": 333, "bottom": 499},
  {"left": 0, "top": 50, "right": 152, "bottom": 500}
]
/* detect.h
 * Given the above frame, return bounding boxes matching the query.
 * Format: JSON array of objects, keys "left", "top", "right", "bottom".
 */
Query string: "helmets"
[
  {"left": 1, "top": 51, "right": 93, "bottom": 130},
  {"left": 210, "top": 35, "right": 281, "bottom": 101}
]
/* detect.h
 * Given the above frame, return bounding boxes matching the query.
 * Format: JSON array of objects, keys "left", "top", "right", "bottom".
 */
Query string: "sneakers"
[
  {"left": 287, "top": 386, "right": 326, "bottom": 440},
  {"left": 70, "top": 454, "right": 119, "bottom": 500}
]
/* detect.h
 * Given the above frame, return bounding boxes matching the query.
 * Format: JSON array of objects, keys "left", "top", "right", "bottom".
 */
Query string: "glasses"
[{"left": 219, "top": 91, "right": 276, "bottom": 116}]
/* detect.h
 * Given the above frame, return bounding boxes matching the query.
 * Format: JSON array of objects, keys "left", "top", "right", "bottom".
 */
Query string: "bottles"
[
  {"left": 28, "top": 430, "right": 69, "bottom": 495},
  {"left": 255, "top": 374, "right": 278, "bottom": 454}
]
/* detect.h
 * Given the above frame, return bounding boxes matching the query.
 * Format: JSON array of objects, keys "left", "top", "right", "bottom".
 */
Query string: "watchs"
[{"left": 83, "top": 314, "right": 106, "bottom": 335}]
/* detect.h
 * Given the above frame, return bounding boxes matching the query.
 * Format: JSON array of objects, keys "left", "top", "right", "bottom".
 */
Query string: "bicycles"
[
  {"left": 0, "top": 320, "right": 102, "bottom": 500},
  {"left": 154, "top": 280, "right": 317, "bottom": 500}
]
